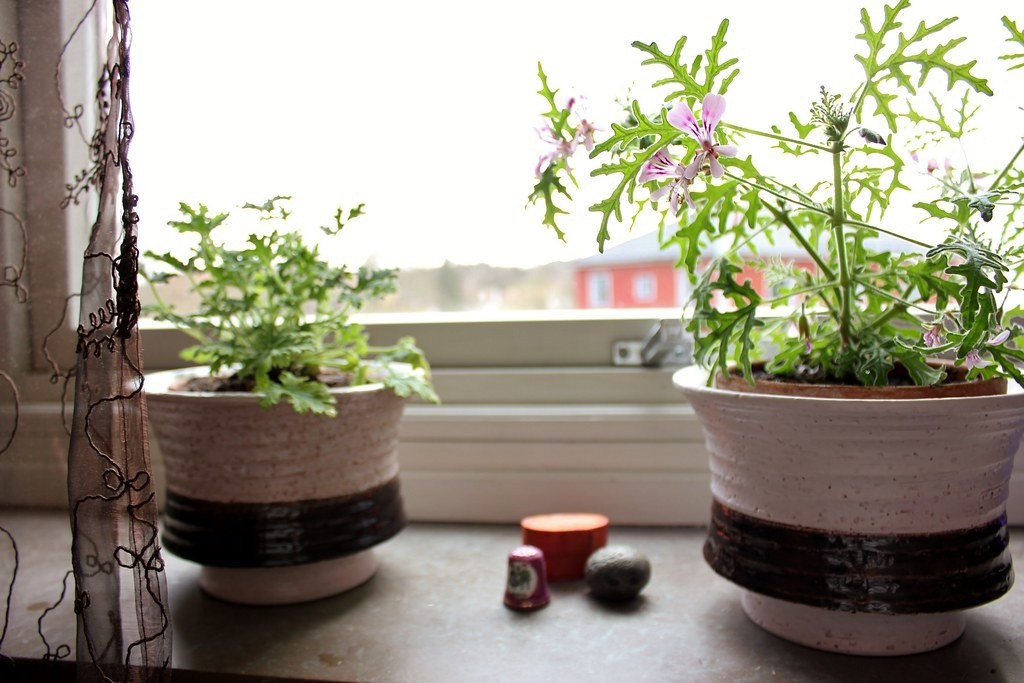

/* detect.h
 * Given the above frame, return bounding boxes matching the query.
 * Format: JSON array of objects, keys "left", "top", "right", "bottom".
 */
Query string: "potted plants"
[{"left": 137, "top": 194, "right": 440, "bottom": 606}]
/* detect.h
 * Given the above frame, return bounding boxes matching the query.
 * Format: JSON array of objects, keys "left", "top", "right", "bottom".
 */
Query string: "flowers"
[{"left": 515, "top": 0, "right": 1023, "bottom": 393}]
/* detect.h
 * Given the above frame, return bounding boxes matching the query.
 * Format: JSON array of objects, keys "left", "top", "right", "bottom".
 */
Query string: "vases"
[{"left": 673, "top": 357, "right": 1023, "bottom": 657}]
